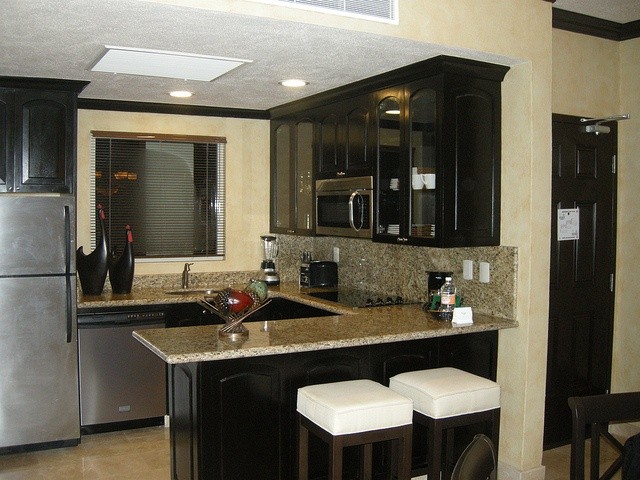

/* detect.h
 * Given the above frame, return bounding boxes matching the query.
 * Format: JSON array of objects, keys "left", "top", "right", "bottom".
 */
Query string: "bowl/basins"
[{"left": 428, "top": 310, "right": 453, "bottom": 321}]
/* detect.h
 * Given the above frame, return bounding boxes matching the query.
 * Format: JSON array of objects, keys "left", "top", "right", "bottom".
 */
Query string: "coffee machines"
[{"left": 422, "top": 268, "right": 452, "bottom": 305}]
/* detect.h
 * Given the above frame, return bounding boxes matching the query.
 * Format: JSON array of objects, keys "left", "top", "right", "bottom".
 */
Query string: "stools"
[
  {"left": 294, "top": 377, "right": 414, "bottom": 480},
  {"left": 388, "top": 366, "right": 500, "bottom": 480}
]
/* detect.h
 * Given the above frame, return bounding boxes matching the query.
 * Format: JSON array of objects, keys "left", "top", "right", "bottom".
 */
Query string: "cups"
[
  {"left": 420, "top": 174, "right": 436, "bottom": 189},
  {"left": 411, "top": 174, "right": 423, "bottom": 190}
]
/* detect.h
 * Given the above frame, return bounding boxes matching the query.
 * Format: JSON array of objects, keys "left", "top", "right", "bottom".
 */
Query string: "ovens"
[{"left": 77, "top": 309, "right": 169, "bottom": 435}]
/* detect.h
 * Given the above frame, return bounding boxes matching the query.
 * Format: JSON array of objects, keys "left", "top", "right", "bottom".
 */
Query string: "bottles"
[
  {"left": 307, "top": 251, "right": 314, "bottom": 264},
  {"left": 298, "top": 253, "right": 311, "bottom": 286},
  {"left": 440, "top": 277, "right": 456, "bottom": 323}
]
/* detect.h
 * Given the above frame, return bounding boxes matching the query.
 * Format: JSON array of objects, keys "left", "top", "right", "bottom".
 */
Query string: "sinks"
[{"left": 165, "top": 291, "right": 222, "bottom": 294}]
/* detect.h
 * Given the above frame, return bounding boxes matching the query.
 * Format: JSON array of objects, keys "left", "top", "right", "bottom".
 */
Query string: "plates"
[
  {"left": 412, "top": 224, "right": 430, "bottom": 235},
  {"left": 387, "top": 225, "right": 399, "bottom": 235},
  {"left": 430, "top": 224, "right": 435, "bottom": 238}
]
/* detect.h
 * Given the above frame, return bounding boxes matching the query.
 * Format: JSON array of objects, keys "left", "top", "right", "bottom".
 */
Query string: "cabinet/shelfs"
[
  {"left": 0, "top": 86, "right": 77, "bottom": 194},
  {"left": 370, "top": 72, "right": 443, "bottom": 240},
  {"left": 315, "top": 94, "right": 370, "bottom": 173},
  {"left": 269, "top": 105, "right": 315, "bottom": 233}
]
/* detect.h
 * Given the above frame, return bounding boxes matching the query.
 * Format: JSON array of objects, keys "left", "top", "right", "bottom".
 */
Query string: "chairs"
[{"left": 567, "top": 391, "right": 640, "bottom": 479}]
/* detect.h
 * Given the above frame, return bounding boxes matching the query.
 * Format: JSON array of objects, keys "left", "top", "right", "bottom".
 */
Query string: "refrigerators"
[{"left": 0, "top": 191, "right": 82, "bottom": 451}]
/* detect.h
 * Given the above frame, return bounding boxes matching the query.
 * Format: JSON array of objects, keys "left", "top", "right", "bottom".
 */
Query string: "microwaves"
[{"left": 316, "top": 176, "right": 373, "bottom": 238}]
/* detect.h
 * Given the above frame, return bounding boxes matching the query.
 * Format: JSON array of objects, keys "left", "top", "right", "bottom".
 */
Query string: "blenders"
[{"left": 254, "top": 235, "right": 281, "bottom": 286}]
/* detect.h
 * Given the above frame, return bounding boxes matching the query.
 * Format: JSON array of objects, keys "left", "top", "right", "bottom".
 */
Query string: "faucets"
[{"left": 182, "top": 263, "right": 193, "bottom": 288}]
